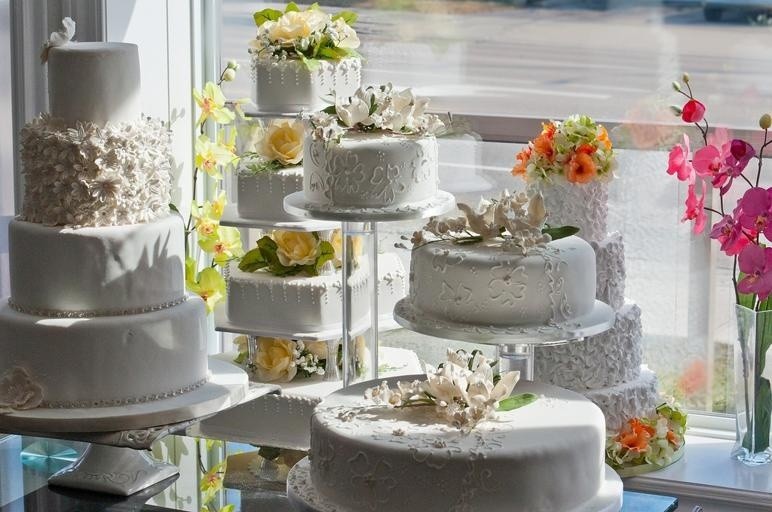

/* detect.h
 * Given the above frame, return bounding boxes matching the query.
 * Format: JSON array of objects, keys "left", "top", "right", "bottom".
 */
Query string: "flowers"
[
  {"left": 161, "top": 58, "right": 252, "bottom": 317},
  {"left": 188, "top": 435, "right": 243, "bottom": 512},
  {"left": 661, "top": 70, "right": 770, "bottom": 452}
]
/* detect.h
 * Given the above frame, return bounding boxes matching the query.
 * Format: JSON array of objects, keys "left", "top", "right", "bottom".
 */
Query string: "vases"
[{"left": 729, "top": 299, "right": 772, "bottom": 469}]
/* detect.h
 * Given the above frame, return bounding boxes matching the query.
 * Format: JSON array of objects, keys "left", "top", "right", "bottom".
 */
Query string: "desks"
[{"left": 0, "top": 433, "right": 681, "bottom": 512}]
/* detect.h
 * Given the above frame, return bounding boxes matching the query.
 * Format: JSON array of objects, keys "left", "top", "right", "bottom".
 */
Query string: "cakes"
[
  {"left": 288, "top": 347, "right": 606, "bottom": 512},
  {"left": 510, "top": 114, "right": 687, "bottom": 469},
  {"left": 0, "top": 16, "right": 209, "bottom": 414},
  {"left": 300, "top": 82, "right": 451, "bottom": 214},
  {"left": 393, "top": 188, "right": 597, "bottom": 335},
  {"left": 200, "top": 2, "right": 374, "bottom": 448}
]
